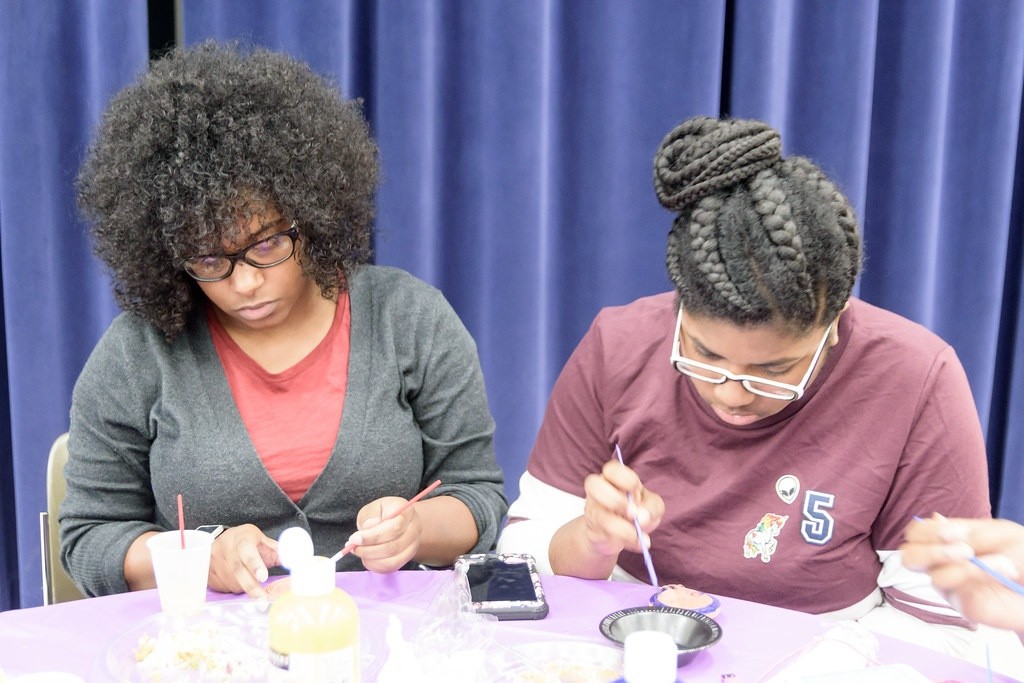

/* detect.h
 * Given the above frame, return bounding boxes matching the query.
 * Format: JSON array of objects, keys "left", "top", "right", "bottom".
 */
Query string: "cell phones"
[{"left": 454, "top": 553, "right": 549, "bottom": 620}]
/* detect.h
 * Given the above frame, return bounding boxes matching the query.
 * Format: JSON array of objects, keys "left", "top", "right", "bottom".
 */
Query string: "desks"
[{"left": 0, "top": 567, "right": 1021, "bottom": 683}]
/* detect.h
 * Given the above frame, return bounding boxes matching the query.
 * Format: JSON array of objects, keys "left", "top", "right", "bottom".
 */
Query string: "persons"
[
  {"left": 59, "top": 38, "right": 508, "bottom": 613},
  {"left": 901, "top": 512, "right": 1024, "bottom": 647},
  {"left": 496, "top": 118, "right": 993, "bottom": 644}
]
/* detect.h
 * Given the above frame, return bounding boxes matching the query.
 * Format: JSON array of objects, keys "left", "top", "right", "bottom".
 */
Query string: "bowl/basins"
[{"left": 598, "top": 606, "right": 723, "bottom": 668}]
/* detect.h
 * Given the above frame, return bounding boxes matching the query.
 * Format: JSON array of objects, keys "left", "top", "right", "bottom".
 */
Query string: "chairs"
[{"left": 39, "top": 432, "right": 86, "bottom": 609}]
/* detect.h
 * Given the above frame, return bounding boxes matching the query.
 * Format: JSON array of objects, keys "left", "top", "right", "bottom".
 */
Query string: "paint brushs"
[
  {"left": 330, "top": 479, "right": 441, "bottom": 561},
  {"left": 912, "top": 514, "right": 1024, "bottom": 597},
  {"left": 615, "top": 443, "right": 659, "bottom": 594}
]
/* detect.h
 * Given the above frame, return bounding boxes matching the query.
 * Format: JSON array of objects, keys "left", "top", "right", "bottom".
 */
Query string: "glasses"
[
  {"left": 668, "top": 298, "right": 833, "bottom": 402},
  {"left": 169, "top": 217, "right": 303, "bottom": 284}
]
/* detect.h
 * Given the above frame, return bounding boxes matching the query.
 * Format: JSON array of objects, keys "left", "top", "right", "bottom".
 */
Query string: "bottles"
[{"left": 266, "top": 526, "right": 361, "bottom": 683}]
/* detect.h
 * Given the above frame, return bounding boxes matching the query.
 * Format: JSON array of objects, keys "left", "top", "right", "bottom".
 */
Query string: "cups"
[
  {"left": 147, "top": 530, "right": 215, "bottom": 611},
  {"left": 623, "top": 631, "right": 677, "bottom": 683}
]
